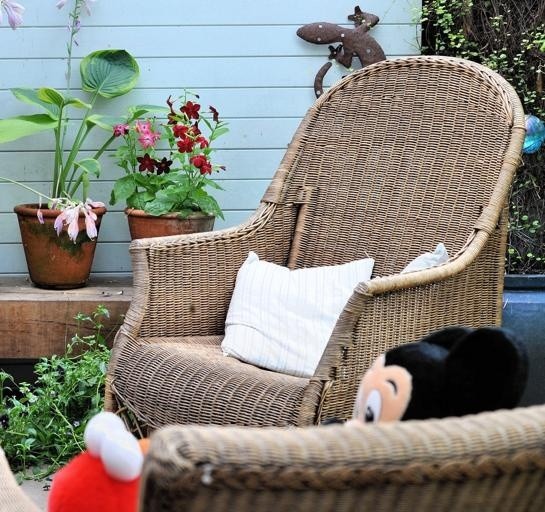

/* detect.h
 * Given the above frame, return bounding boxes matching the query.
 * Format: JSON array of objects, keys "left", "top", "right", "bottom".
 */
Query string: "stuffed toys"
[
  {"left": 48, "top": 411, "right": 157, "bottom": 511},
  {"left": 323, "top": 323, "right": 530, "bottom": 424}
]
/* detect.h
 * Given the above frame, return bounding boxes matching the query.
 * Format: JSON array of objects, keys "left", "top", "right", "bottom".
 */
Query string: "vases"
[
  {"left": 12, "top": 202, "right": 108, "bottom": 291},
  {"left": 124, "top": 207, "right": 219, "bottom": 241}
]
